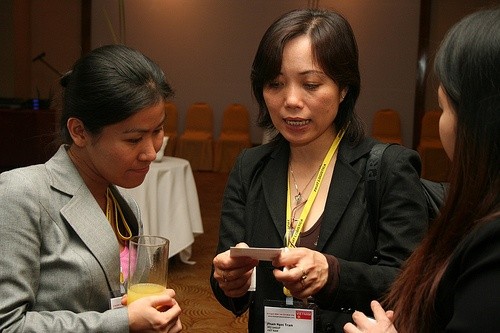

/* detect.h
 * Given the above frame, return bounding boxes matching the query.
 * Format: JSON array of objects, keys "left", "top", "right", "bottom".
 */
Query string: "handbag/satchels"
[{"left": 365, "top": 142, "right": 455, "bottom": 222}]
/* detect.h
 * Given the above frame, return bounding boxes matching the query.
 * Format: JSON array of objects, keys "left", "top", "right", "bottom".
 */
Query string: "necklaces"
[{"left": 289, "top": 160, "right": 320, "bottom": 201}]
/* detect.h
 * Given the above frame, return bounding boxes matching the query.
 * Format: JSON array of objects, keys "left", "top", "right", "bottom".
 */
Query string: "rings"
[
  {"left": 299, "top": 275, "right": 307, "bottom": 288},
  {"left": 221, "top": 270, "right": 227, "bottom": 282}
]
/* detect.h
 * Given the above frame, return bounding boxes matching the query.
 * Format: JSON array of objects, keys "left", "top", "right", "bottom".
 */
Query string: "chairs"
[
  {"left": 176, "top": 103, "right": 213, "bottom": 171},
  {"left": 214, "top": 103, "right": 252, "bottom": 172},
  {"left": 164, "top": 101, "right": 178, "bottom": 155},
  {"left": 371, "top": 109, "right": 449, "bottom": 182}
]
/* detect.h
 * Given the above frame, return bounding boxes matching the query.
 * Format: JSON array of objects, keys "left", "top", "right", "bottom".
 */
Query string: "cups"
[{"left": 127, "top": 236, "right": 170, "bottom": 309}]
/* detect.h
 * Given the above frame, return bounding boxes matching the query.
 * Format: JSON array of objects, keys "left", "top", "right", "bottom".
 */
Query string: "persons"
[
  {"left": 208, "top": 8, "right": 430, "bottom": 333},
  {"left": 0, "top": 43, "right": 183, "bottom": 333},
  {"left": 342, "top": 9, "right": 500, "bottom": 332}
]
